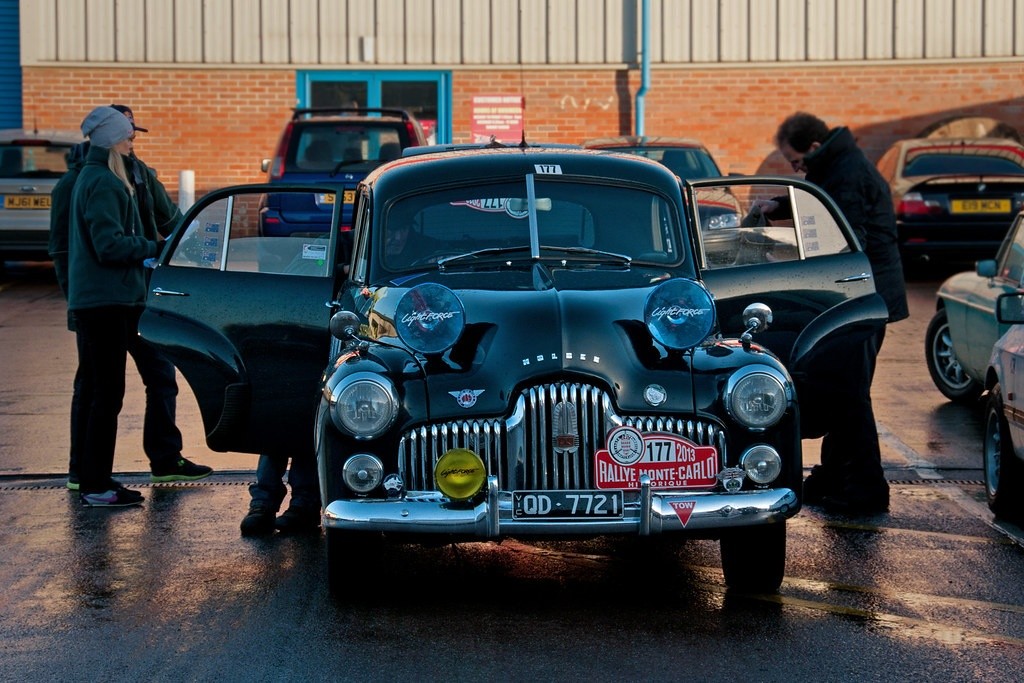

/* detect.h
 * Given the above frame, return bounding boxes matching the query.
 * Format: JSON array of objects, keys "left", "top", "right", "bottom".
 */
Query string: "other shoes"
[
  {"left": 273, "top": 507, "right": 321, "bottom": 530},
  {"left": 241, "top": 502, "right": 276, "bottom": 530}
]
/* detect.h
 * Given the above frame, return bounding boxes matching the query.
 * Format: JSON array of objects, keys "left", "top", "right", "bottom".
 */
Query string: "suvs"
[{"left": 254, "top": 108, "right": 430, "bottom": 270}]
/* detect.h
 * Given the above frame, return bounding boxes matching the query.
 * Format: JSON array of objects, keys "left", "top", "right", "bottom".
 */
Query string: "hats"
[
  {"left": 108, "top": 103, "right": 148, "bottom": 132},
  {"left": 80, "top": 106, "right": 134, "bottom": 148}
]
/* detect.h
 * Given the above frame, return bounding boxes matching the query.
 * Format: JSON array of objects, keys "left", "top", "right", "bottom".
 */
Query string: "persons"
[
  {"left": 749, "top": 112, "right": 908, "bottom": 518},
  {"left": 240, "top": 216, "right": 420, "bottom": 536},
  {"left": 49, "top": 105, "right": 214, "bottom": 506}
]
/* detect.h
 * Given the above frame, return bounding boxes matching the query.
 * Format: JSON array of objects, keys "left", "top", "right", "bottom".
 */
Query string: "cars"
[
  {"left": 924, "top": 211, "right": 1024, "bottom": 406},
  {"left": 577, "top": 138, "right": 757, "bottom": 267},
  {"left": 137, "top": 141, "right": 891, "bottom": 597},
  {"left": 0, "top": 130, "right": 98, "bottom": 277},
  {"left": 973, "top": 285, "right": 1023, "bottom": 518},
  {"left": 872, "top": 137, "right": 1023, "bottom": 277}
]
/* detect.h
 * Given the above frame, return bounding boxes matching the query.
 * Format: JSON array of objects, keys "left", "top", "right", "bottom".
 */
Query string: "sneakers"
[
  {"left": 66, "top": 476, "right": 121, "bottom": 490},
  {"left": 80, "top": 486, "right": 145, "bottom": 508},
  {"left": 150, "top": 458, "right": 213, "bottom": 482}
]
